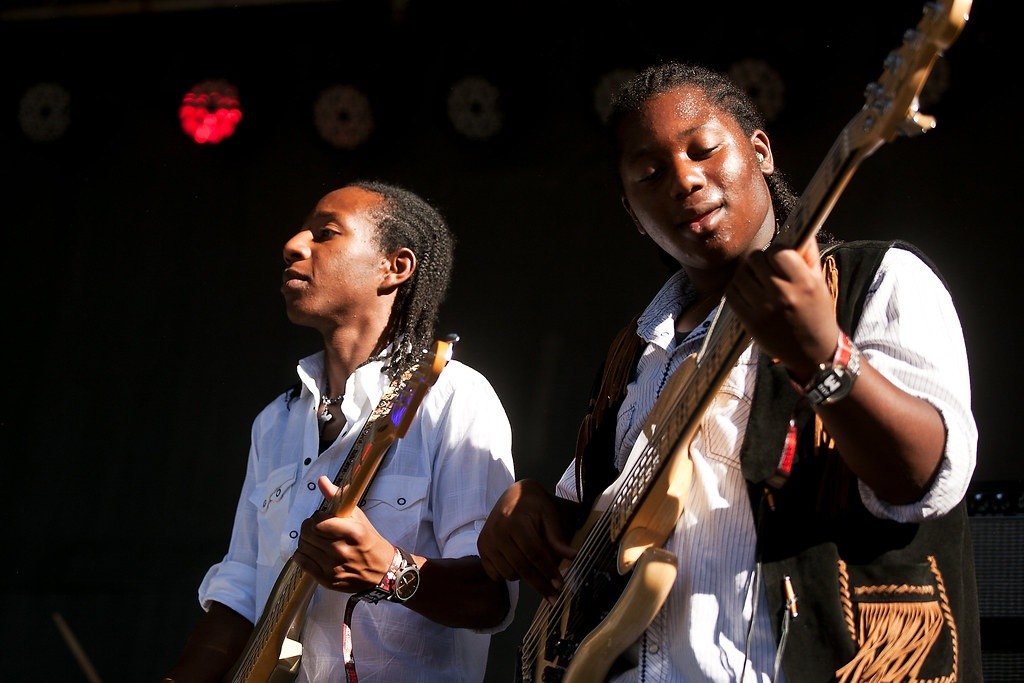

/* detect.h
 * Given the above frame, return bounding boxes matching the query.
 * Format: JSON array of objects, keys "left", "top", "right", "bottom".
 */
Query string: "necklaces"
[{"left": 318, "top": 393, "right": 344, "bottom": 426}]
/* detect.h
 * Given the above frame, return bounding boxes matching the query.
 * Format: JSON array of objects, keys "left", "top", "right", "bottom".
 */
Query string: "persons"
[
  {"left": 477, "top": 63, "right": 979, "bottom": 683},
  {"left": 161, "top": 181, "right": 521, "bottom": 683}
]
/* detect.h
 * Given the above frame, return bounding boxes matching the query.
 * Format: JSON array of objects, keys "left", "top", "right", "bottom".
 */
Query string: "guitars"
[
  {"left": 518, "top": 0, "right": 976, "bottom": 683},
  {"left": 225, "top": 330, "right": 463, "bottom": 683}
]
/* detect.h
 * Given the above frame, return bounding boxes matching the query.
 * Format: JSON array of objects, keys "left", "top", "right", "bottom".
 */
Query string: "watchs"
[
  {"left": 786, "top": 330, "right": 862, "bottom": 405},
  {"left": 383, "top": 546, "right": 420, "bottom": 605}
]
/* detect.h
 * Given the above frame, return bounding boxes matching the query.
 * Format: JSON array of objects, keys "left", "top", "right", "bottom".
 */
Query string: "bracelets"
[
  {"left": 790, "top": 331, "right": 850, "bottom": 404},
  {"left": 342, "top": 543, "right": 404, "bottom": 683}
]
